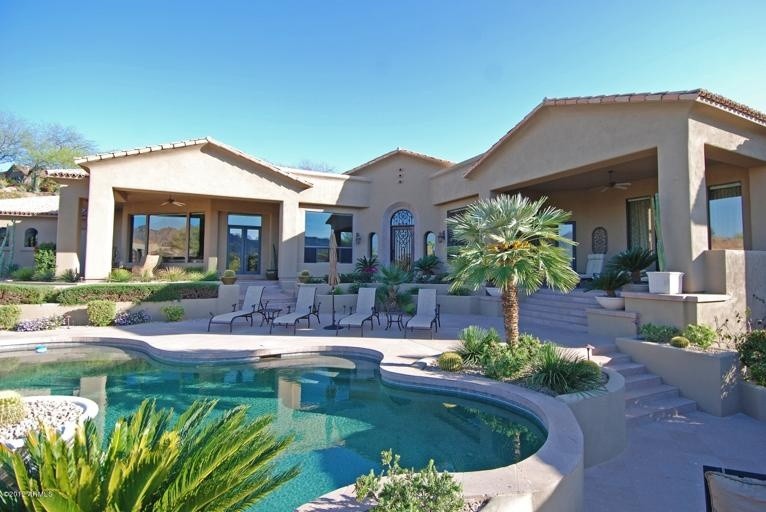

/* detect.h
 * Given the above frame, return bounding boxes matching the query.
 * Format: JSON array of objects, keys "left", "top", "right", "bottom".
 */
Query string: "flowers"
[{"left": 354, "top": 255, "right": 380, "bottom": 275}]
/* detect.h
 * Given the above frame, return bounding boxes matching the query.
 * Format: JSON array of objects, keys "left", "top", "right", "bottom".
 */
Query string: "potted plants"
[
  {"left": 221, "top": 270, "right": 238, "bottom": 285},
  {"left": 298, "top": 269, "right": 313, "bottom": 283},
  {"left": 414, "top": 255, "right": 443, "bottom": 283},
  {"left": 583, "top": 245, "right": 658, "bottom": 310}
]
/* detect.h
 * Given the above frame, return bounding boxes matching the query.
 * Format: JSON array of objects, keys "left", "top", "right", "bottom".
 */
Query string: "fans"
[
  {"left": 591, "top": 169, "right": 631, "bottom": 193},
  {"left": 159, "top": 194, "right": 186, "bottom": 207}
]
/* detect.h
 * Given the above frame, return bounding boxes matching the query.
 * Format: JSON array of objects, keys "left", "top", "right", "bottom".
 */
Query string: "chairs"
[
  {"left": 207, "top": 284, "right": 440, "bottom": 339},
  {"left": 576, "top": 253, "right": 605, "bottom": 289}
]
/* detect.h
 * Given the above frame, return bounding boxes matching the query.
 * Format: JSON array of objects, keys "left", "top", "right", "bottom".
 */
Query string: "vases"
[{"left": 645, "top": 271, "right": 683, "bottom": 293}]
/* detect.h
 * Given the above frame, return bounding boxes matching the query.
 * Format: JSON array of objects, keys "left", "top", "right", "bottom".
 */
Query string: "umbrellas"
[{"left": 322, "top": 228, "right": 348, "bottom": 330}]
